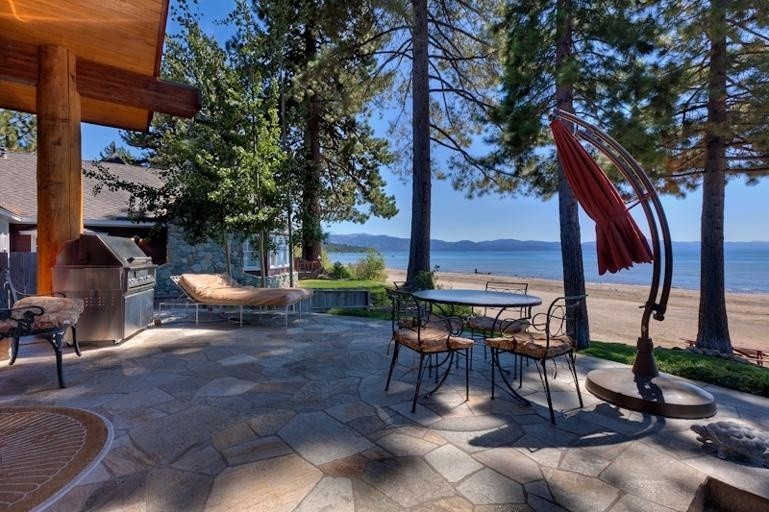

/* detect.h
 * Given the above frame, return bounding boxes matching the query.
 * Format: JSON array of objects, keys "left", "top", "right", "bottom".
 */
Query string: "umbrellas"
[{"left": 549, "top": 109, "right": 674, "bottom": 375}]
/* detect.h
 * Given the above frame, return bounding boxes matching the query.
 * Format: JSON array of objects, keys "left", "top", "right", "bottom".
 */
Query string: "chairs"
[
  {"left": 1, "top": 267, "right": 85, "bottom": 390},
  {"left": 383, "top": 283, "right": 590, "bottom": 424},
  {"left": 159, "top": 272, "right": 315, "bottom": 334}
]
